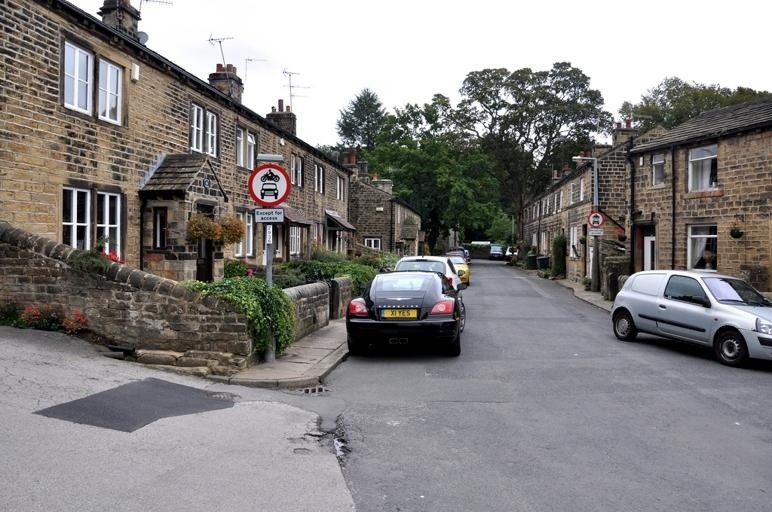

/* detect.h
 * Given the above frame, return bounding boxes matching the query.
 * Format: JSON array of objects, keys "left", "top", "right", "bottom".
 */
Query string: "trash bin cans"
[{"left": 527, "top": 255, "right": 549, "bottom": 270}]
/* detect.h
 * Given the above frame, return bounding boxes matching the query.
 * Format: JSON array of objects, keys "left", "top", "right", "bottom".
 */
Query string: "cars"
[
  {"left": 346, "top": 247, "right": 472, "bottom": 357},
  {"left": 488, "top": 244, "right": 518, "bottom": 260},
  {"left": 611, "top": 270, "right": 772, "bottom": 367}
]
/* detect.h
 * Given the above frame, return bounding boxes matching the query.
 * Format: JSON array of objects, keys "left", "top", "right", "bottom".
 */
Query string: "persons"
[{"left": 698, "top": 250, "right": 717, "bottom": 268}]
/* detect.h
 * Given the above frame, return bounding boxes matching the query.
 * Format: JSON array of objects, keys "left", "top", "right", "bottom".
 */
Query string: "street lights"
[
  {"left": 572, "top": 156, "right": 600, "bottom": 291},
  {"left": 256, "top": 154, "right": 285, "bottom": 291}
]
